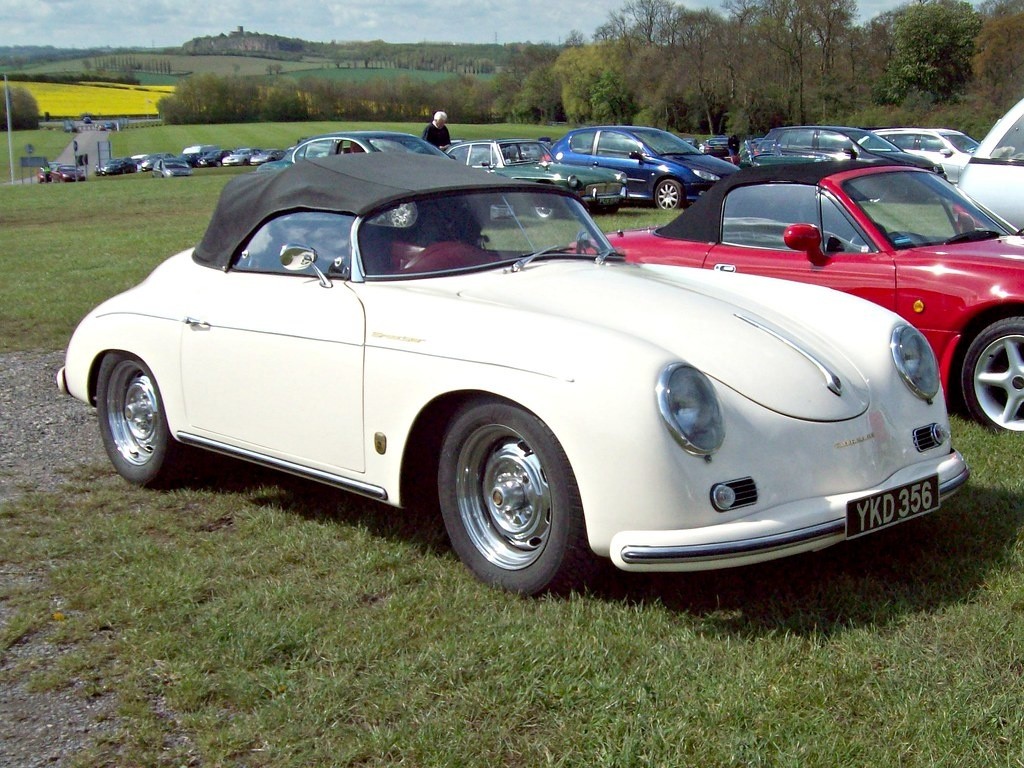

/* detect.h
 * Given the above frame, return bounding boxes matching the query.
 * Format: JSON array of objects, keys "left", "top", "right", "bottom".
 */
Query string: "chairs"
[{"left": 350, "top": 142, "right": 364, "bottom": 154}]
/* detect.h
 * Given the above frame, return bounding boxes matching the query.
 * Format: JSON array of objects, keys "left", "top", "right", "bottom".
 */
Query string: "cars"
[
  {"left": 445, "top": 138, "right": 631, "bottom": 222},
  {"left": 252, "top": 129, "right": 467, "bottom": 228},
  {"left": 38, "top": 142, "right": 331, "bottom": 184},
  {"left": 853, "top": 126, "right": 981, "bottom": 171},
  {"left": 739, "top": 123, "right": 947, "bottom": 184},
  {"left": 80, "top": 116, "right": 114, "bottom": 131},
  {"left": 936, "top": 97, "right": 1024, "bottom": 235},
  {"left": 538, "top": 124, "right": 743, "bottom": 210},
  {"left": 683, "top": 134, "right": 784, "bottom": 167},
  {"left": 567, "top": 157, "right": 1024, "bottom": 441},
  {"left": 56, "top": 149, "right": 971, "bottom": 599}
]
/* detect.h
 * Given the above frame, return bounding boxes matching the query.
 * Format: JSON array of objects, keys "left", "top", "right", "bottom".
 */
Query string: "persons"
[
  {"left": 422, "top": 111, "right": 452, "bottom": 151},
  {"left": 728, "top": 135, "right": 740, "bottom": 156}
]
